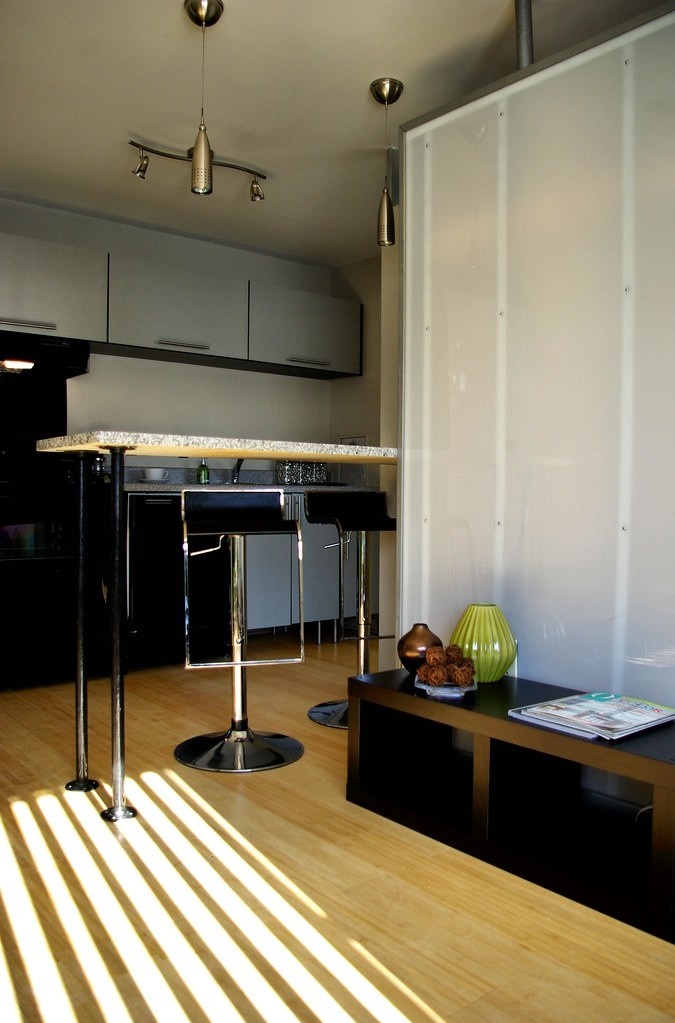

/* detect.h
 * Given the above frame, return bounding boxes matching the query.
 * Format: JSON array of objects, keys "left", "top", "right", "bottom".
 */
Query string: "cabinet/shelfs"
[
  {"left": 346, "top": 668, "right": 675, "bottom": 945},
  {"left": 244, "top": 485, "right": 379, "bottom": 644},
  {"left": 0, "top": 232, "right": 364, "bottom": 380}
]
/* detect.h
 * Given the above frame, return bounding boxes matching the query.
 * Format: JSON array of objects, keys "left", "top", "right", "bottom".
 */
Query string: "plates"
[
  {"left": 414, "top": 675, "right": 478, "bottom": 698},
  {"left": 139, "top": 479, "right": 169, "bottom": 483}
]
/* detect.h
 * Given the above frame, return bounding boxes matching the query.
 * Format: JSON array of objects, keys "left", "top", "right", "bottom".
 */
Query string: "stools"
[
  {"left": 172, "top": 487, "right": 306, "bottom": 774},
  {"left": 305, "top": 490, "right": 398, "bottom": 730}
]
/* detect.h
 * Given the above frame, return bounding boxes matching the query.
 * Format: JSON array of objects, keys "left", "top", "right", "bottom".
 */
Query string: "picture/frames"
[{"left": 338, "top": 434, "right": 369, "bottom": 484}]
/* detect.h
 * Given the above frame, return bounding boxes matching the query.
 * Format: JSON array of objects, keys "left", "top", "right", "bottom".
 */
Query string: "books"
[{"left": 508, "top": 690, "right": 675, "bottom": 741}]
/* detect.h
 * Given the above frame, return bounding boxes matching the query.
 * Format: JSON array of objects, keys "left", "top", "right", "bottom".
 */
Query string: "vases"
[
  {"left": 398, "top": 623, "right": 444, "bottom": 675},
  {"left": 450, "top": 602, "right": 518, "bottom": 684}
]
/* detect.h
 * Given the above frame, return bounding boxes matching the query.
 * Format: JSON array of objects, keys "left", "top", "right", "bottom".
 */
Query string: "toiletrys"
[{"left": 197, "top": 458, "right": 210, "bottom": 484}]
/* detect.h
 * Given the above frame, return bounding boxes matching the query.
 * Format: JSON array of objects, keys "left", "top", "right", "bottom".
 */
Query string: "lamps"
[
  {"left": 370, "top": 78, "right": 404, "bottom": 246},
  {"left": 127, "top": 0, "right": 267, "bottom": 202}
]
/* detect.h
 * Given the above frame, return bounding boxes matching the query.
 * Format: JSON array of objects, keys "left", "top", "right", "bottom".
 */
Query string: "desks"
[{"left": 36, "top": 430, "right": 400, "bottom": 821}]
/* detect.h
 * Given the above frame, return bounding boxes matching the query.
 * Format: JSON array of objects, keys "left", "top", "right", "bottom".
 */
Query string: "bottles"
[{"left": 197, "top": 458, "right": 210, "bottom": 484}]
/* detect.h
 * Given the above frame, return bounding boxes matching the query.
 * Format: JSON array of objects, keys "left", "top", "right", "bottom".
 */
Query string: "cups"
[{"left": 144, "top": 469, "right": 168, "bottom": 479}]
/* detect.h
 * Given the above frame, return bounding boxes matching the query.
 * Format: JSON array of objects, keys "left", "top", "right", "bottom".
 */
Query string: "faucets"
[{"left": 233, "top": 459, "right": 244, "bottom": 483}]
